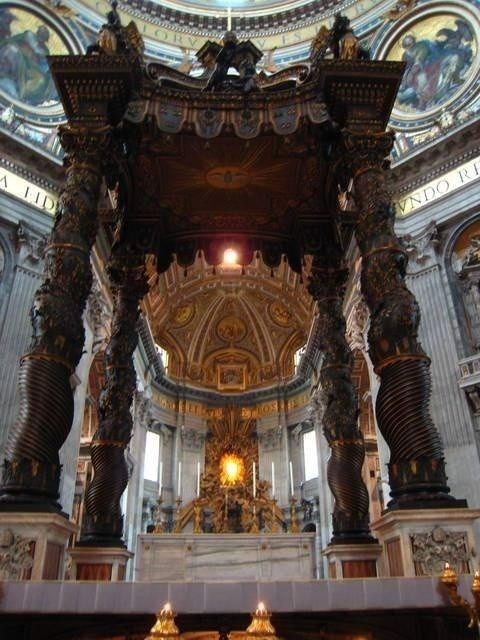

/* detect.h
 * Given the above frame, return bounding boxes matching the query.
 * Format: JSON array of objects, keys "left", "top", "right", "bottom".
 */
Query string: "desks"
[{"left": 132, "top": 533, "right": 318, "bottom": 583}]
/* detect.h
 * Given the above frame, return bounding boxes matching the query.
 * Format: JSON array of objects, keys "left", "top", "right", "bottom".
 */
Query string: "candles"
[
  {"left": 290, "top": 462, "right": 294, "bottom": 496},
  {"left": 252, "top": 462, "right": 255, "bottom": 498},
  {"left": 196, "top": 462, "right": 200, "bottom": 498},
  {"left": 158, "top": 461, "right": 163, "bottom": 498},
  {"left": 271, "top": 462, "right": 275, "bottom": 496},
  {"left": 178, "top": 461, "right": 181, "bottom": 496}
]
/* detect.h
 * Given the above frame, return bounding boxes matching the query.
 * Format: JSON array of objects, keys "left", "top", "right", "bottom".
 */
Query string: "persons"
[
  {"left": 396, "top": 34, "right": 459, "bottom": 114},
  {"left": 192, "top": 501, "right": 203, "bottom": 533},
  {"left": 0, "top": 23, "right": 53, "bottom": 102}
]
